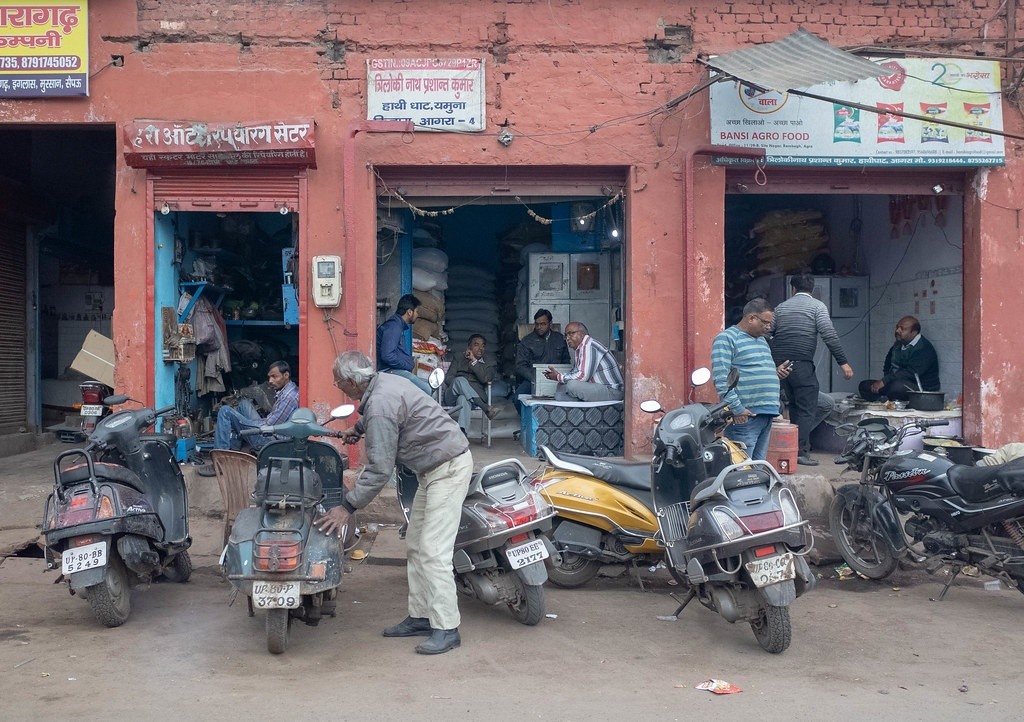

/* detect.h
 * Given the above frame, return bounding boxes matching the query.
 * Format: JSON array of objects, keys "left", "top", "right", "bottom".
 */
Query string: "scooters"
[
  {"left": 41, "top": 395, "right": 191, "bottom": 627},
  {"left": 528, "top": 443, "right": 666, "bottom": 593},
  {"left": 396, "top": 458, "right": 558, "bottom": 626},
  {"left": 640, "top": 368, "right": 816, "bottom": 653},
  {"left": 218, "top": 404, "right": 365, "bottom": 654}
]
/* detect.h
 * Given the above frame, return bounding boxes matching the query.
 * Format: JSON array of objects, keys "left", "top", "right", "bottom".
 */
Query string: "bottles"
[
  {"left": 49, "top": 307, "right": 56, "bottom": 316},
  {"left": 223, "top": 312, "right": 232, "bottom": 320},
  {"left": 175, "top": 420, "right": 190, "bottom": 439},
  {"left": 233, "top": 310, "right": 240, "bottom": 320},
  {"left": 62, "top": 313, "right": 101, "bottom": 321}
]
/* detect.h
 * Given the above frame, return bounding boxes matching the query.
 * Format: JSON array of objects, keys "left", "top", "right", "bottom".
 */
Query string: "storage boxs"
[
  {"left": 531, "top": 364, "right": 573, "bottom": 400},
  {"left": 69, "top": 329, "right": 115, "bottom": 388}
]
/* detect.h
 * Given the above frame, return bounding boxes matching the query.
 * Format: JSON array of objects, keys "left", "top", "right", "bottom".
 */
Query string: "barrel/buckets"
[{"left": 766, "top": 422, "right": 799, "bottom": 473}]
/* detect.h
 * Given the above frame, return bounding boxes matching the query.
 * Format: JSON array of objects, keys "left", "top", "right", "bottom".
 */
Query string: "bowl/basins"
[
  {"left": 893, "top": 401, "right": 909, "bottom": 410},
  {"left": 971, "top": 448, "right": 996, "bottom": 461}
]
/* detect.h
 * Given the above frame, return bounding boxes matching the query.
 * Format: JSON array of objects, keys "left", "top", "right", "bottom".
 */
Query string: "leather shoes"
[
  {"left": 382, "top": 615, "right": 433, "bottom": 636},
  {"left": 414, "top": 627, "right": 461, "bottom": 653}
]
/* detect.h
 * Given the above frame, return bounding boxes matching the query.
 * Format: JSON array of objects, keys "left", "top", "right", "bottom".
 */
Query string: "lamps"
[{"left": 568, "top": 202, "right": 598, "bottom": 232}]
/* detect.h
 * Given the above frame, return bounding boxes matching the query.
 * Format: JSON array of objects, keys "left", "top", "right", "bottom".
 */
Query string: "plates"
[{"left": 855, "top": 404, "right": 887, "bottom": 411}]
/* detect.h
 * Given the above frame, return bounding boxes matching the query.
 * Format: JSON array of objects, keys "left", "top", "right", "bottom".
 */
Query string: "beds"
[
  {"left": 517, "top": 394, "right": 625, "bottom": 458},
  {"left": 811, "top": 402, "right": 964, "bottom": 461}
]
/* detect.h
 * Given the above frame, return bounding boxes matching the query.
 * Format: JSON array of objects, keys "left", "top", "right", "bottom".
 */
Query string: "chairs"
[
  {"left": 436, "top": 357, "right": 492, "bottom": 448},
  {"left": 209, "top": 448, "right": 259, "bottom": 548}
]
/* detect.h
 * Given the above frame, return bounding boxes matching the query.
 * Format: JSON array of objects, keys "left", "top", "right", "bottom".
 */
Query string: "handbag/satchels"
[
  {"left": 196, "top": 333, "right": 220, "bottom": 354},
  {"left": 192, "top": 296, "right": 214, "bottom": 345}
]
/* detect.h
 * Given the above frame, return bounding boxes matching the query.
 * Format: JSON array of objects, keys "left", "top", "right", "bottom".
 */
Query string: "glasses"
[
  {"left": 333, "top": 377, "right": 346, "bottom": 388},
  {"left": 563, "top": 331, "right": 582, "bottom": 340},
  {"left": 753, "top": 315, "right": 772, "bottom": 327}
]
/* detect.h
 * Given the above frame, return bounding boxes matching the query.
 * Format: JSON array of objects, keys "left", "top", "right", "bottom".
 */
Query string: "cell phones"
[{"left": 783, "top": 361, "right": 794, "bottom": 371}]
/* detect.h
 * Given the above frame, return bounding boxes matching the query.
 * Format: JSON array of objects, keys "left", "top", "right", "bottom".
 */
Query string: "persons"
[
  {"left": 711, "top": 297, "right": 793, "bottom": 470},
  {"left": 197, "top": 361, "right": 299, "bottom": 477},
  {"left": 543, "top": 322, "right": 625, "bottom": 401},
  {"left": 859, "top": 316, "right": 941, "bottom": 403},
  {"left": 443, "top": 334, "right": 502, "bottom": 435},
  {"left": 377, "top": 294, "right": 432, "bottom": 395},
  {"left": 313, "top": 350, "right": 473, "bottom": 654},
  {"left": 512, "top": 308, "right": 571, "bottom": 418},
  {"left": 765, "top": 274, "right": 853, "bottom": 466}
]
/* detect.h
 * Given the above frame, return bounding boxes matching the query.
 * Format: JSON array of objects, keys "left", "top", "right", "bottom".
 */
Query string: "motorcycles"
[
  {"left": 73, "top": 382, "right": 115, "bottom": 437},
  {"left": 830, "top": 414, "right": 1024, "bottom": 601}
]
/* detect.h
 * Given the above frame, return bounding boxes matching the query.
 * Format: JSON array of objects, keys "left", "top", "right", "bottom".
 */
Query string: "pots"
[{"left": 907, "top": 391, "right": 945, "bottom": 411}]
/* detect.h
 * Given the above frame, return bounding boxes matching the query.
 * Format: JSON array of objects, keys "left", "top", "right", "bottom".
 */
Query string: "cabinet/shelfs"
[{"left": 771, "top": 274, "right": 870, "bottom": 395}]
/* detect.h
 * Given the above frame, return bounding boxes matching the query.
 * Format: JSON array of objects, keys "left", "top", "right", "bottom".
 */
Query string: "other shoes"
[
  {"left": 198, "top": 464, "right": 222, "bottom": 476},
  {"left": 798, "top": 456, "right": 819, "bottom": 465},
  {"left": 486, "top": 406, "right": 501, "bottom": 420}
]
